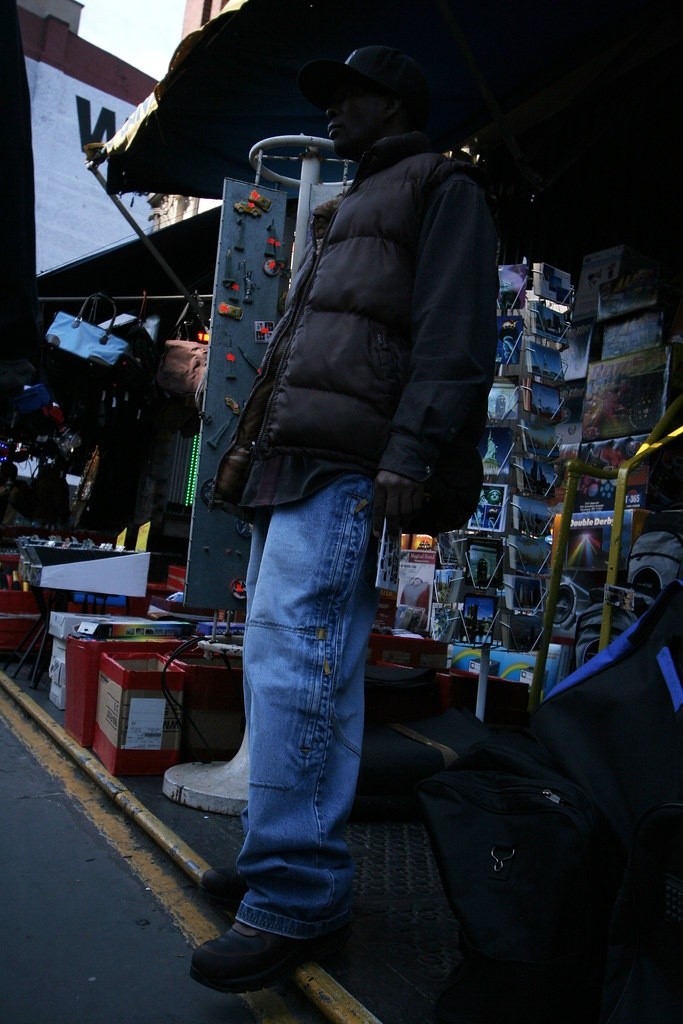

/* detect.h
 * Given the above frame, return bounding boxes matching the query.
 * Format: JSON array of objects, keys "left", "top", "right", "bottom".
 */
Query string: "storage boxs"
[
  {"left": 164, "top": 651, "right": 246, "bottom": 765},
  {"left": 374, "top": 587, "right": 396, "bottom": 629},
  {"left": 377, "top": 661, "right": 530, "bottom": 727},
  {"left": 0, "top": 527, "right": 248, "bottom": 710},
  {"left": 65, "top": 633, "right": 198, "bottom": 749},
  {"left": 91, "top": 652, "right": 184, "bottom": 777},
  {"left": 366, "top": 634, "right": 448, "bottom": 668}
]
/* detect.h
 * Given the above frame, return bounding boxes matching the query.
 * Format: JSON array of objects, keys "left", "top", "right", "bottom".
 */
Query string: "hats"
[{"left": 297, "top": 45, "right": 430, "bottom": 132}]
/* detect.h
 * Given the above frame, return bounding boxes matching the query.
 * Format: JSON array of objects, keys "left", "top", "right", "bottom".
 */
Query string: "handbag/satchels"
[
  {"left": 0, "top": 290, "right": 211, "bottom": 526},
  {"left": 415, "top": 581, "right": 683, "bottom": 1024}
]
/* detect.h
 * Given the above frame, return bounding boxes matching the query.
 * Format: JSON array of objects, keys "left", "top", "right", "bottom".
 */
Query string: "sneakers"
[
  {"left": 204, "top": 865, "right": 248, "bottom": 908},
  {"left": 190, "top": 921, "right": 351, "bottom": 994}
]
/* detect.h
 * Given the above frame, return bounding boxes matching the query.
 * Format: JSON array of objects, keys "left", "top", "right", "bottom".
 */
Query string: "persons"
[
  {"left": 0, "top": 463, "right": 32, "bottom": 525},
  {"left": 189, "top": 46, "right": 498, "bottom": 992}
]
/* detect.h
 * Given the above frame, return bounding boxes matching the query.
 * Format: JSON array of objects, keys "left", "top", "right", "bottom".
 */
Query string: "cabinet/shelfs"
[{"left": 180, "top": 131, "right": 683, "bottom": 686}]
